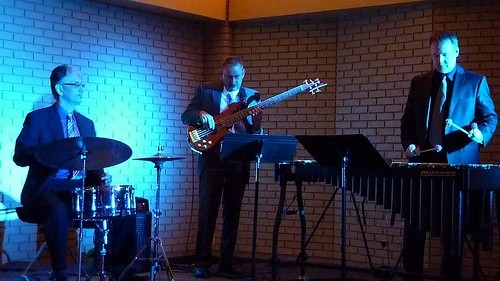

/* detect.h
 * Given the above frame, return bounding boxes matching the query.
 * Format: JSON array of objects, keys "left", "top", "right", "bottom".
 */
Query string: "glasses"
[{"left": 62, "top": 83, "right": 86, "bottom": 88}]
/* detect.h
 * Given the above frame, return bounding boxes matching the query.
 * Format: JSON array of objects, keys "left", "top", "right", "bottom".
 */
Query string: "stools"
[{"left": 15, "top": 207, "right": 91, "bottom": 281}]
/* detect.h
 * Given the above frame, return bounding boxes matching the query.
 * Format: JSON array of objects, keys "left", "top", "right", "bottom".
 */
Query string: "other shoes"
[
  {"left": 194, "top": 263, "right": 209, "bottom": 278},
  {"left": 215, "top": 267, "right": 239, "bottom": 279}
]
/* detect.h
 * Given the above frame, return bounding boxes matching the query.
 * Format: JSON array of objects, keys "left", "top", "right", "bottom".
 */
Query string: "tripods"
[{"left": 118, "top": 165, "right": 175, "bottom": 281}]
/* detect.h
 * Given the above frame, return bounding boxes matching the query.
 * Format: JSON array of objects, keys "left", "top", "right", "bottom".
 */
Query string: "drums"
[{"left": 71, "top": 184, "right": 138, "bottom": 219}]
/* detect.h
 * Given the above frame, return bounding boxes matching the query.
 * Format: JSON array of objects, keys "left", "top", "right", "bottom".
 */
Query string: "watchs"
[{"left": 251, "top": 128, "right": 261, "bottom": 134}]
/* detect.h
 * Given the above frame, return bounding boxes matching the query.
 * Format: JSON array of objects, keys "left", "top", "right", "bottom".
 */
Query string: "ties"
[
  {"left": 67, "top": 114, "right": 81, "bottom": 177},
  {"left": 429, "top": 75, "right": 448, "bottom": 146}
]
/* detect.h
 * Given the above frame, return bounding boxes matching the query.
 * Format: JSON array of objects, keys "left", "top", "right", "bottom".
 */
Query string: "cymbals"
[
  {"left": 133, "top": 153, "right": 186, "bottom": 168},
  {"left": 36, "top": 136, "right": 132, "bottom": 170}
]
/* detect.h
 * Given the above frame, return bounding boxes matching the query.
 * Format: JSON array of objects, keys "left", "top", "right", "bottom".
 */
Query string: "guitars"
[{"left": 188, "top": 77, "right": 328, "bottom": 151}]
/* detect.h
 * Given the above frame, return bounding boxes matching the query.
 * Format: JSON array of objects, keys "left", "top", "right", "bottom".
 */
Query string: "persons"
[
  {"left": 181, "top": 57, "right": 263, "bottom": 281},
  {"left": 401, "top": 30, "right": 498, "bottom": 281},
  {"left": 13, "top": 65, "right": 150, "bottom": 280}
]
